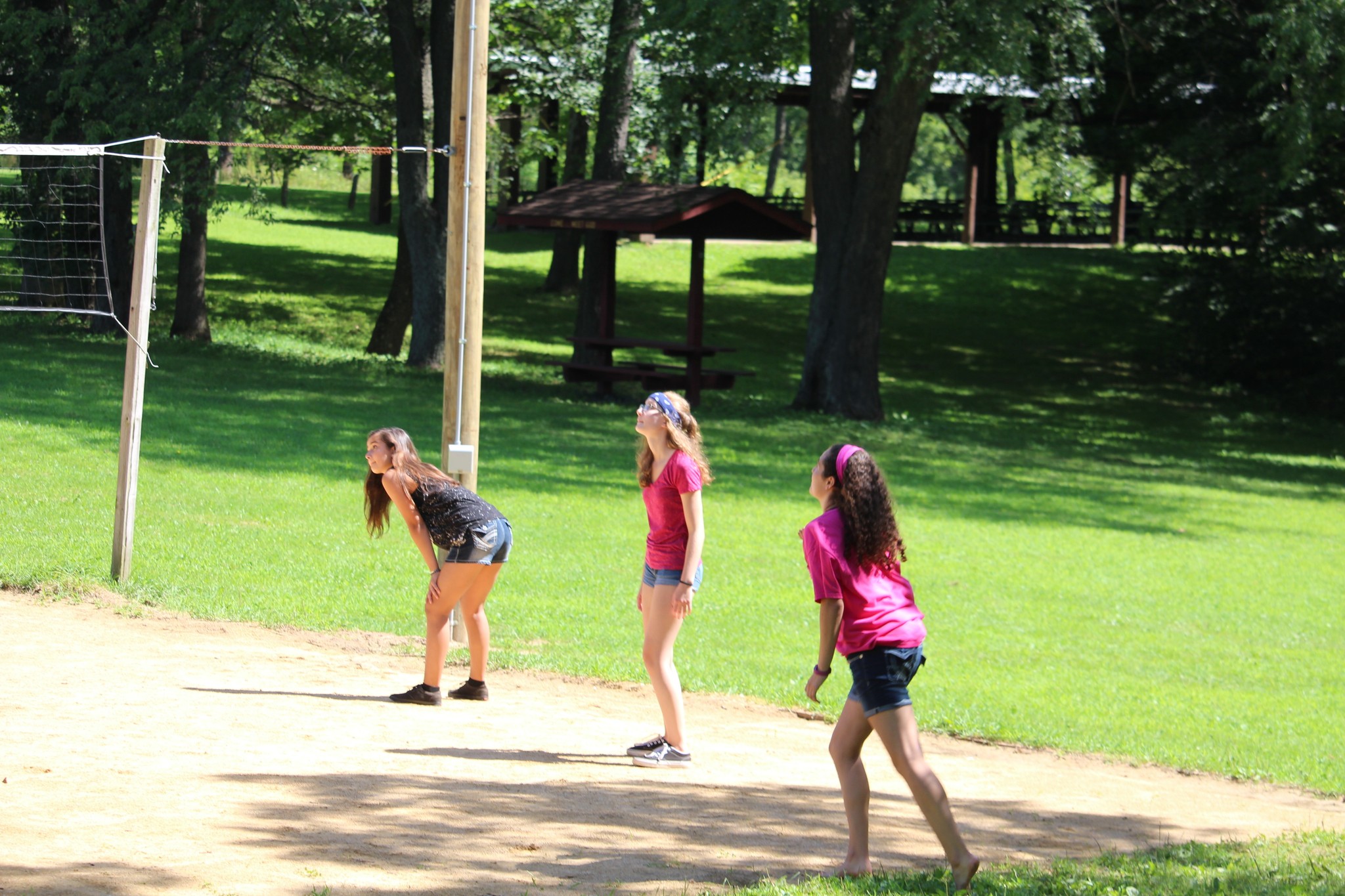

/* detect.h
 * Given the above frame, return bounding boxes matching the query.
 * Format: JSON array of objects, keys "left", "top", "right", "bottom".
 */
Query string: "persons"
[
  {"left": 626, "top": 390, "right": 715, "bottom": 770},
  {"left": 362, "top": 426, "right": 513, "bottom": 706},
  {"left": 799, "top": 444, "right": 980, "bottom": 889}
]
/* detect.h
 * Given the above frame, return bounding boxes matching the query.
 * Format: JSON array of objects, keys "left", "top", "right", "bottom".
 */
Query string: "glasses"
[{"left": 639, "top": 402, "right": 662, "bottom": 414}]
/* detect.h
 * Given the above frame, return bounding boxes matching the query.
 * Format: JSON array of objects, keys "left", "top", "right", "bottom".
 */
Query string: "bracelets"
[
  {"left": 430, "top": 569, "right": 441, "bottom": 575},
  {"left": 680, "top": 580, "right": 693, "bottom": 586},
  {"left": 814, "top": 665, "right": 832, "bottom": 675}
]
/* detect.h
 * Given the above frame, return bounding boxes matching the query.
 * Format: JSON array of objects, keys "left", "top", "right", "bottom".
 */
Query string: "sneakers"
[
  {"left": 449, "top": 681, "right": 489, "bottom": 700},
  {"left": 628, "top": 734, "right": 675, "bottom": 756},
  {"left": 632, "top": 743, "right": 691, "bottom": 768},
  {"left": 389, "top": 684, "right": 442, "bottom": 705}
]
